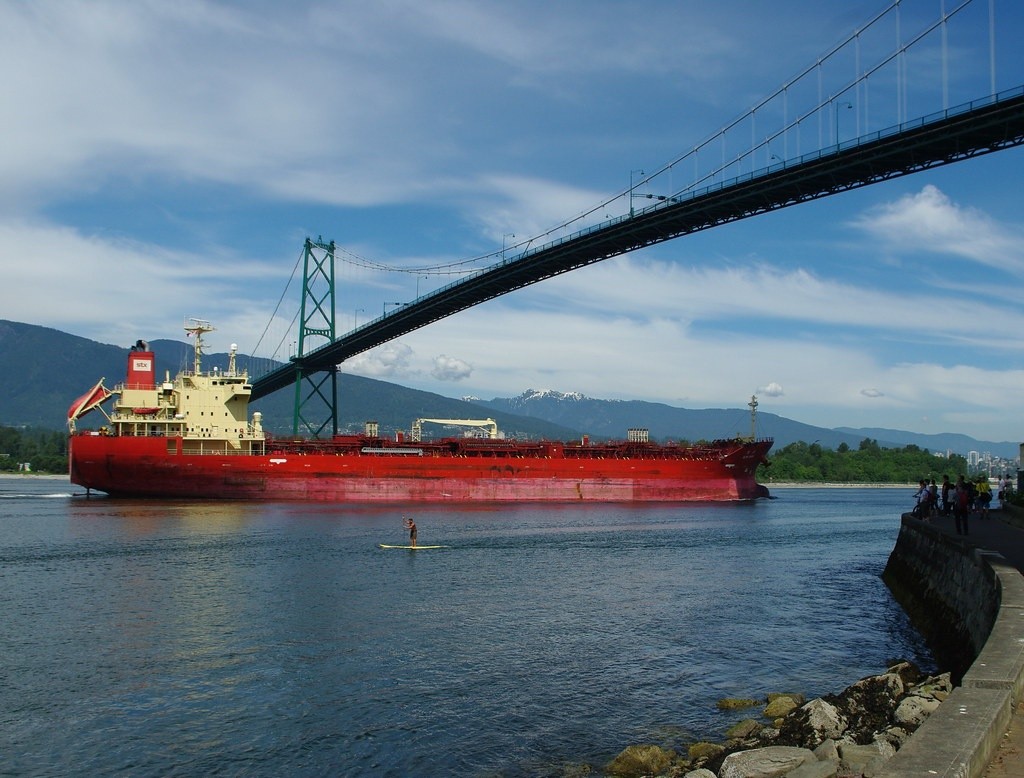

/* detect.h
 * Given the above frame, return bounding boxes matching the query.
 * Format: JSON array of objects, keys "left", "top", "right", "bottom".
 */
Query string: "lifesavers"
[
  {"left": 239, "top": 428, "right": 244, "bottom": 439},
  {"left": 159, "top": 432, "right": 165, "bottom": 436}
]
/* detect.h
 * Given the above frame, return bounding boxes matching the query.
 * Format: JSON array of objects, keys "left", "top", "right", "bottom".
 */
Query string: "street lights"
[
  {"left": 416, "top": 276, "right": 427, "bottom": 303},
  {"left": 502, "top": 233, "right": 515, "bottom": 265},
  {"left": 771, "top": 154, "right": 785, "bottom": 171},
  {"left": 836, "top": 100, "right": 853, "bottom": 151},
  {"left": 289, "top": 343, "right": 292, "bottom": 358},
  {"left": 629, "top": 169, "right": 644, "bottom": 219},
  {"left": 354, "top": 308, "right": 364, "bottom": 331},
  {"left": 293, "top": 341, "right": 297, "bottom": 356}
]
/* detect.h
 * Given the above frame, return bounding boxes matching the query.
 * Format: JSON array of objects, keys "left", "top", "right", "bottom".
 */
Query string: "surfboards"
[{"left": 375, "top": 542, "right": 454, "bottom": 550}]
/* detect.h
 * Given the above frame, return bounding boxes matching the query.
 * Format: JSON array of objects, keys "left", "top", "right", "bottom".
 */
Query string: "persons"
[
  {"left": 402, "top": 517, "right": 418, "bottom": 547},
  {"left": 913, "top": 473, "right": 1013, "bottom": 534}
]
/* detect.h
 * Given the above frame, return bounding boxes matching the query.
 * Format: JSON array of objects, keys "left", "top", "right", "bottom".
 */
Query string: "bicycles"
[{"left": 912, "top": 493, "right": 951, "bottom": 519}]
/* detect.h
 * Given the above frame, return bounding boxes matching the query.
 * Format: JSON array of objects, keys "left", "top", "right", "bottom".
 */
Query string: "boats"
[{"left": 65, "top": 315, "right": 776, "bottom": 502}]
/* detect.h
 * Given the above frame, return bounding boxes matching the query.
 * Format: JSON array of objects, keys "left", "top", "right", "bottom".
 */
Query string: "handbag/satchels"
[{"left": 981, "top": 491, "right": 992, "bottom": 502}]
